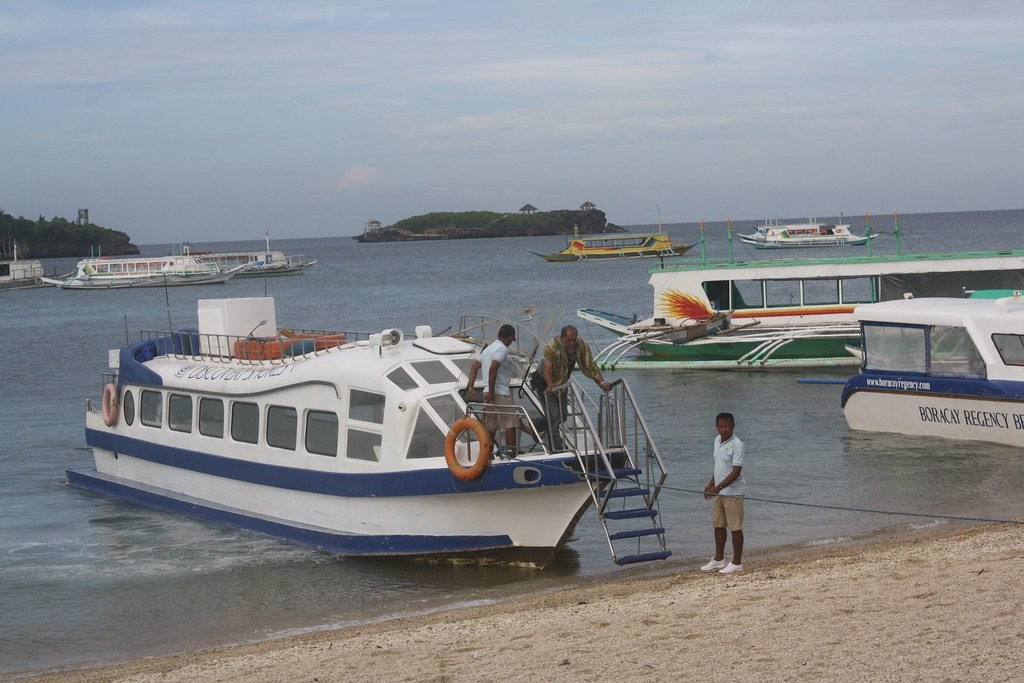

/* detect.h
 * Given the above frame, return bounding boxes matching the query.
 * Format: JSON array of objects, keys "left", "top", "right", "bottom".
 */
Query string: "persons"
[
  {"left": 467, "top": 324, "right": 527, "bottom": 460},
  {"left": 528, "top": 325, "right": 611, "bottom": 452},
  {"left": 699, "top": 413, "right": 748, "bottom": 574}
]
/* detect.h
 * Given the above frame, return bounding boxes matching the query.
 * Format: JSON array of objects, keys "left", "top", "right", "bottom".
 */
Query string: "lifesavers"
[{"left": 444, "top": 418, "right": 492, "bottom": 480}]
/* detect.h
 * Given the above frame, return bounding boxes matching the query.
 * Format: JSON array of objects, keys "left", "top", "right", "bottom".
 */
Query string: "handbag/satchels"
[{"left": 528, "top": 370, "right": 547, "bottom": 391}]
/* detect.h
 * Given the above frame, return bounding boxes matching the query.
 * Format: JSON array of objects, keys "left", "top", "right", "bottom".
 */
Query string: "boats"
[
  {"left": 178, "top": 244, "right": 316, "bottom": 279},
  {"left": 739, "top": 221, "right": 790, "bottom": 242},
  {"left": 1, "top": 244, "right": 46, "bottom": 284},
  {"left": 790, "top": 283, "right": 1023, "bottom": 449},
  {"left": 740, "top": 226, "right": 880, "bottom": 246},
  {"left": 526, "top": 222, "right": 700, "bottom": 263},
  {"left": 572, "top": 241, "right": 1023, "bottom": 367},
  {"left": 61, "top": 273, "right": 671, "bottom": 575},
  {"left": 40, "top": 242, "right": 246, "bottom": 289}
]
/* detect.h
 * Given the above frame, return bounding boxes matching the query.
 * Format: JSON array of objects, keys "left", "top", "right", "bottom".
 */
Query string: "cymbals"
[{"left": 102, "top": 383, "right": 119, "bottom": 427}]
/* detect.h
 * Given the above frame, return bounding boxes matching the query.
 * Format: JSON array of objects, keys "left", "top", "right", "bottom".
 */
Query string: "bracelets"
[
  {"left": 598, "top": 381, "right": 604, "bottom": 387},
  {"left": 717, "top": 483, "right": 725, "bottom": 491}
]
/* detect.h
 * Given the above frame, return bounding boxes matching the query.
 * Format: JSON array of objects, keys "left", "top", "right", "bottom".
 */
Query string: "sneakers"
[
  {"left": 700, "top": 558, "right": 726, "bottom": 572},
  {"left": 719, "top": 562, "right": 744, "bottom": 575}
]
